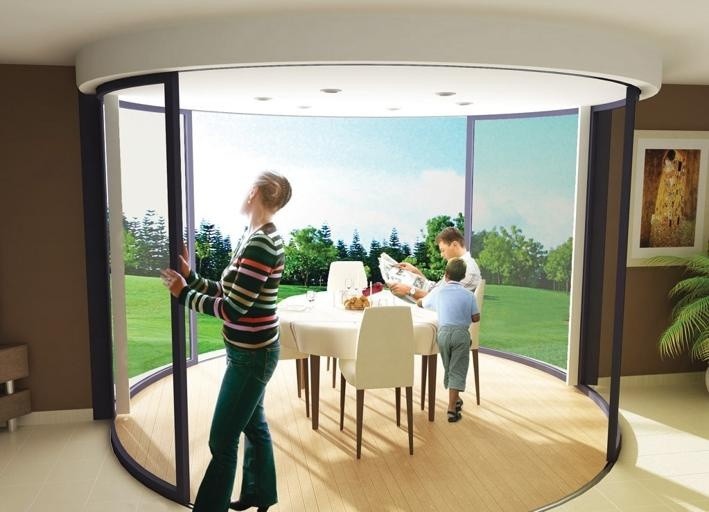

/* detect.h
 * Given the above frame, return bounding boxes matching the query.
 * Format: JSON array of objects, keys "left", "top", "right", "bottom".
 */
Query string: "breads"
[{"left": 344, "top": 296, "right": 369, "bottom": 310}]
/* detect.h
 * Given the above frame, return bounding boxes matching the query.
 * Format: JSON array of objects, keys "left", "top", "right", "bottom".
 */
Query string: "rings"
[{"left": 167, "top": 278, "right": 172, "bottom": 281}]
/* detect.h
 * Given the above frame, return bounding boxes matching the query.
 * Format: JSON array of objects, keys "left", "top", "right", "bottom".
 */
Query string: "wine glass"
[{"left": 306, "top": 291, "right": 316, "bottom": 314}]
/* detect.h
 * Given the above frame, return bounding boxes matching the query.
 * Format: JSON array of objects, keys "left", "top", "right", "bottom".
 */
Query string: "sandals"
[
  {"left": 447, "top": 411, "right": 461, "bottom": 422},
  {"left": 456, "top": 398, "right": 463, "bottom": 412}
]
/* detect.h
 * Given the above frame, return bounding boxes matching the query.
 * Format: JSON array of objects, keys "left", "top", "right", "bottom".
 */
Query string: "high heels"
[{"left": 229, "top": 500, "right": 270, "bottom": 512}]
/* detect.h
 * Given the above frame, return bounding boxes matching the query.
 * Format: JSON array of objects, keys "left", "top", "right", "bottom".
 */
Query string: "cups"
[{"left": 345, "top": 278, "right": 352, "bottom": 289}]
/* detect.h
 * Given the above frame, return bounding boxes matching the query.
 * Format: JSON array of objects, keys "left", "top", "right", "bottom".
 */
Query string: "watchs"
[{"left": 409, "top": 286, "right": 415, "bottom": 297}]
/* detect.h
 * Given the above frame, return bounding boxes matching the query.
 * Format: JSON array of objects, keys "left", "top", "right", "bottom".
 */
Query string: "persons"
[
  {"left": 158, "top": 169, "right": 295, "bottom": 512},
  {"left": 415, "top": 255, "right": 480, "bottom": 424},
  {"left": 388, "top": 227, "right": 483, "bottom": 301}
]
